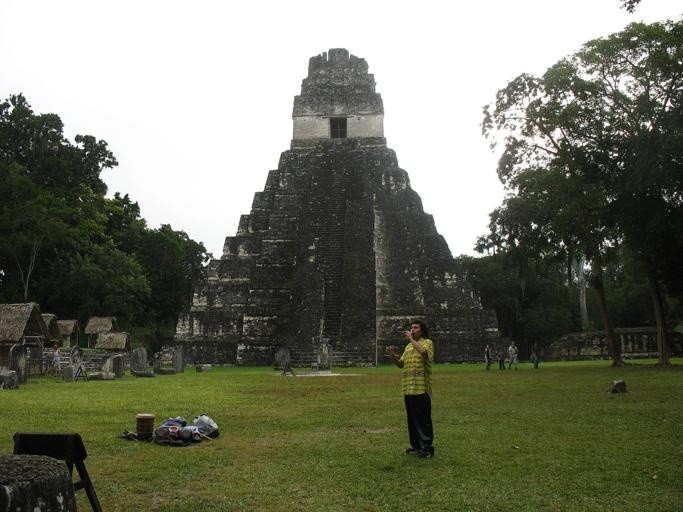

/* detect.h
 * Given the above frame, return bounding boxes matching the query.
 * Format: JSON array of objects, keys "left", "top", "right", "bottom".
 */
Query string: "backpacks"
[
  {"left": 156, "top": 415, "right": 187, "bottom": 437},
  {"left": 194, "top": 415, "right": 219, "bottom": 439}
]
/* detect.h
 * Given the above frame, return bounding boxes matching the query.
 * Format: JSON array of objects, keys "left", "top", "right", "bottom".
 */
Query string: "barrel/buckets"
[{"left": 136, "top": 414, "right": 154, "bottom": 438}]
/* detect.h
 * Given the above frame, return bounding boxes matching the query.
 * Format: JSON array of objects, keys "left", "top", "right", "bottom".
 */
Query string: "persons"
[
  {"left": 530, "top": 342, "right": 539, "bottom": 368},
  {"left": 508, "top": 340, "right": 518, "bottom": 370},
  {"left": 496, "top": 344, "right": 506, "bottom": 370},
  {"left": 283, "top": 357, "right": 297, "bottom": 376},
  {"left": 51, "top": 345, "right": 61, "bottom": 374},
  {"left": 388, "top": 319, "right": 435, "bottom": 459},
  {"left": 484, "top": 344, "right": 492, "bottom": 370}
]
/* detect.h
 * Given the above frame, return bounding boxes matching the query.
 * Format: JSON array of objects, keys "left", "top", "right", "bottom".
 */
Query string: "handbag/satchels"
[{"left": 155, "top": 428, "right": 191, "bottom": 446}]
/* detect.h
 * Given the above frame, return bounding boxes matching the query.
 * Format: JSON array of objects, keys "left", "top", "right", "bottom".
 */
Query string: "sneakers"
[
  {"left": 422, "top": 448, "right": 433, "bottom": 458},
  {"left": 407, "top": 448, "right": 421, "bottom": 454}
]
